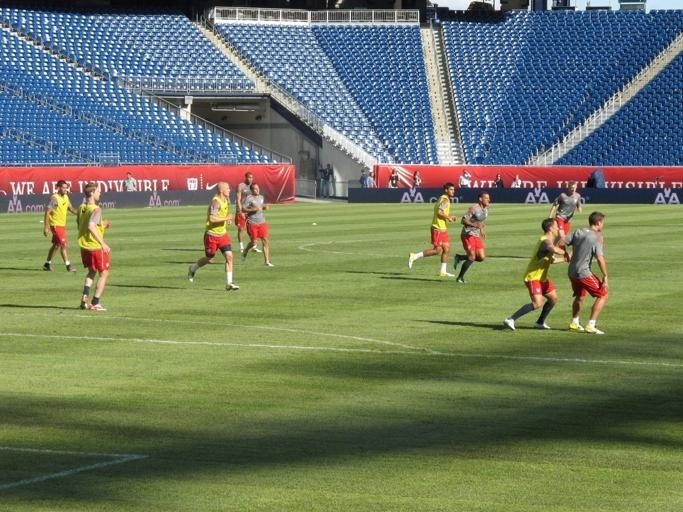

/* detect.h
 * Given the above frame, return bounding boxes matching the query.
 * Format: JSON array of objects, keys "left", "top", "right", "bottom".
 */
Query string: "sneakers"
[
  {"left": 79, "top": 293, "right": 108, "bottom": 313},
  {"left": 582, "top": 322, "right": 608, "bottom": 336},
  {"left": 236, "top": 243, "right": 276, "bottom": 267},
  {"left": 407, "top": 252, "right": 415, "bottom": 269},
  {"left": 567, "top": 322, "right": 585, "bottom": 333},
  {"left": 502, "top": 317, "right": 518, "bottom": 331},
  {"left": 223, "top": 281, "right": 240, "bottom": 292},
  {"left": 186, "top": 264, "right": 197, "bottom": 285},
  {"left": 41, "top": 261, "right": 79, "bottom": 272},
  {"left": 438, "top": 271, "right": 471, "bottom": 284},
  {"left": 452, "top": 253, "right": 460, "bottom": 269},
  {"left": 532, "top": 321, "right": 553, "bottom": 330}
]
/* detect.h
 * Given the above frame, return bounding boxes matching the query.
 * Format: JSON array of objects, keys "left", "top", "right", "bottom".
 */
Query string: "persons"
[
  {"left": 321, "top": 163, "right": 334, "bottom": 198},
  {"left": 187, "top": 181, "right": 240, "bottom": 290},
  {"left": 75, "top": 184, "right": 113, "bottom": 312},
  {"left": 494, "top": 174, "right": 503, "bottom": 188},
  {"left": 558, "top": 212, "right": 608, "bottom": 335},
  {"left": 42, "top": 180, "right": 79, "bottom": 272},
  {"left": 407, "top": 182, "right": 457, "bottom": 277},
  {"left": 454, "top": 192, "right": 491, "bottom": 283},
  {"left": 122, "top": 172, "right": 137, "bottom": 193},
  {"left": 510, "top": 175, "right": 522, "bottom": 189},
  {"left": 412, "top": 171, "right": 421, "bottom": 189},
  {"left": 548, "top": 180, "right": 583, "bottom": 261},
  {"left": 387, "top": 169, "right": 400, "bottom": 188},
  {"left": 503, "top": 218, "right": 572, "bottom": 331},
  {"left": 458, "top": 169, "right": 471, "bottom": 189},
  {"left": 235, "top": 172, "right": 262, "bottom": 253},
  {"left": 240, "top": 182, "right": 275, "bottom": 268},
  {"left": 359, "top": 169, "right": 375, "bottom": 189}
]
